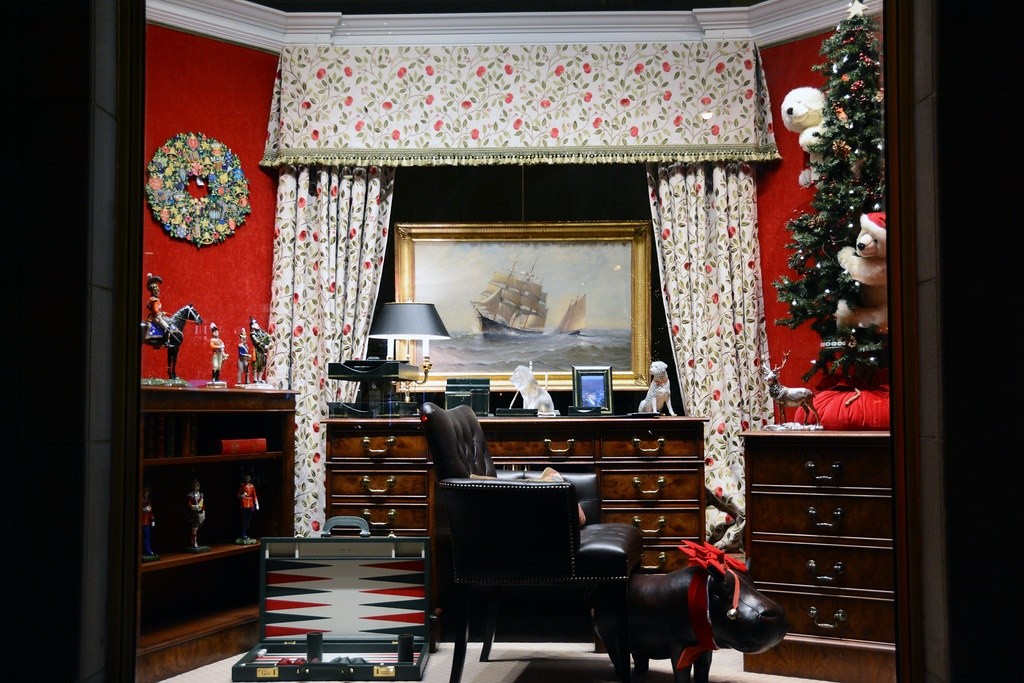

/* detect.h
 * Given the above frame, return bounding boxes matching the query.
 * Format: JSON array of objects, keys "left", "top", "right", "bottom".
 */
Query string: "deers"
[{"left": 752, "top": 350, "right": 821, "bottom": 427}]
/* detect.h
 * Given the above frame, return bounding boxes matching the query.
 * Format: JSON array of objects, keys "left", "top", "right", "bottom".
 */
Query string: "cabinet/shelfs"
[
  {"left": 319, "top": 416, "right": 710, "bottom": 653},
  {"left": 134, "top": 384, "right": 301, "bottom": 683},
  {"left": 738, "top": 428, "right": 896, "bottom": 683}
]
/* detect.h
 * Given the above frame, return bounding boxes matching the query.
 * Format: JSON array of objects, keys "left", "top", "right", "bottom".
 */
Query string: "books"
[{"left": 144, "top": 413, "right": 197, "bottom": 458}]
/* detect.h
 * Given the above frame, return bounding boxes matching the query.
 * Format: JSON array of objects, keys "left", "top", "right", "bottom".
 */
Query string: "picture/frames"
[
  {"left": 572, "top": 364, "right": 614, "bottom": 416},
  {"left": 394, "top": 220, "right": 653, "bottom": 392}
]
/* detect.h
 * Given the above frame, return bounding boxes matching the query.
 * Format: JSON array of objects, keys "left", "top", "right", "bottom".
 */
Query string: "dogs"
[{"left": 638, "top": 361, "right": 677, "bottom": 417}]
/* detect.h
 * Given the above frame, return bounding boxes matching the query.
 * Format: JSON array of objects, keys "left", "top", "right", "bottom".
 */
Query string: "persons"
[
  {"left": 237, "top": 328, "right": 252, "bottom": 384},
  {"left": 187, "top": 479, "right": 205, "bottom": 547},
  {"left": 250, "top": 318, "right": 274, "bottom": 384},
  {"left": 210, "top": 323, "right": 229, "bottom": 382},
  {"left": 142, "top": 487, "right": 156, "bottom": 556},
  {"left": 237, "top": 472, "right": 259, "bottom": 540},
  {"left": 146, "top": 273, "right": 174, "bottom": 347}
]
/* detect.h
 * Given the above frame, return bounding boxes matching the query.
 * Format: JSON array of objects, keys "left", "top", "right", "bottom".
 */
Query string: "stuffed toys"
[
  {"left": 781, "top": 86, "right": 826, "bottom": 189},
  {"left": 835, "top": 213, "right": 889, "bottom": 333}
]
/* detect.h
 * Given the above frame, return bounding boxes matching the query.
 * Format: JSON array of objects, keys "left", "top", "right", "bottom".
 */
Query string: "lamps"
[{"left": 369, "top": 301, "right": 452, "bottom": 414}]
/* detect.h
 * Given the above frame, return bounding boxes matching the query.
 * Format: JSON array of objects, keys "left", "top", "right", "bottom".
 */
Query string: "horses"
[{"left": 141, "top": 304, "right": 203, "bottom": 380}]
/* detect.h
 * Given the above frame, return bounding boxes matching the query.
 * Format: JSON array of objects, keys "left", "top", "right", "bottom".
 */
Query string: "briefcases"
[{"left": 231, "top": 515, "right": 431, "bottom": 682}]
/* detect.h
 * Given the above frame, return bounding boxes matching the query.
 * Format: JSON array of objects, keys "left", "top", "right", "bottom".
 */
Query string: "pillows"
[{"left": 469, "top": 466, "right": 587, "bottom": 528}]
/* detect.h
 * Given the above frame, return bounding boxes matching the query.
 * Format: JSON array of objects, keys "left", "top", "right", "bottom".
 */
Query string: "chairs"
[{"left": 417, "top": 401, "right": 641, "bottom": 683}]
